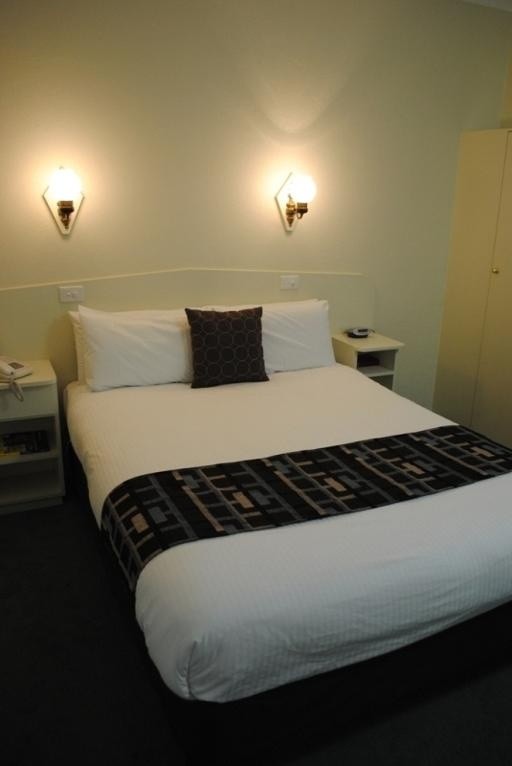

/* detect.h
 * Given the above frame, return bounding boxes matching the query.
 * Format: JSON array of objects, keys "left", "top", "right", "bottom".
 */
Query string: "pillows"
[{"left": 65, "top": 296, "right": 335, "bottom": 392}]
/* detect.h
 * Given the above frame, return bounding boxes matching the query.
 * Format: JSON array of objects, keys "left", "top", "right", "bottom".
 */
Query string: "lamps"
[
  {"left": 43, "top": 165, "right": 86, "bottom": 241},
  {"left": 275, "top": 172, "right": 315, "bottom": 232}
]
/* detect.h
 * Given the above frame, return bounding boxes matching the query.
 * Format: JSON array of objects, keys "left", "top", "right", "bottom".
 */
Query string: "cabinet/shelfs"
[{"left": 432, "top": 128, "right": 512, "bottom": 448}]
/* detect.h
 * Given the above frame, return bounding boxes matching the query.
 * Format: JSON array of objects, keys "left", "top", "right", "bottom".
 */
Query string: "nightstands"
[
  {"left": 0, "top": 361, "right": 67, "bottom": 517},
  {"left": 332, "top": 331, "right": 406, "bottom": 392}
]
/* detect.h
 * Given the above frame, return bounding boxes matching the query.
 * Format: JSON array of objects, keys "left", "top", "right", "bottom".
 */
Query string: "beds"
[{"left": 62, "top": 362, "right": 512, "bottom": 702}]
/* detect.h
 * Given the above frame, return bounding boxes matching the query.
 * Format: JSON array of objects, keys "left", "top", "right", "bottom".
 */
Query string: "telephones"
[{"left": 0, "top": 356, "right": 32, "bottom": 382}]
